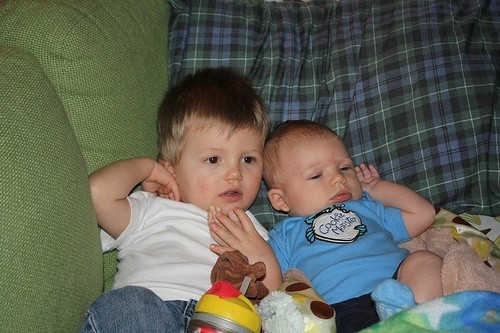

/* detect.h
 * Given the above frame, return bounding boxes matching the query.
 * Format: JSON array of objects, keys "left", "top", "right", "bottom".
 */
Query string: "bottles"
[{"left": 185, "top": 275, "right": 263, "bottom": 333}]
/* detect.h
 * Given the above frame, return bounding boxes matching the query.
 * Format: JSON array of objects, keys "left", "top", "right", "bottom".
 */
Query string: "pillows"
[
  {"left": 0, "top": 0, "right": 168, "bottom": 279},
  {"left": 2, "top": 44, "right": 108, "bottom": 333},
  {"left": 167, "top": 0, "right": 499, "bottom": 229}
]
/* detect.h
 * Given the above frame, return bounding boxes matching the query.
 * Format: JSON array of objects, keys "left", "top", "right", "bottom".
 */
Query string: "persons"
[
  {"left": 208, "top": 118, "right": 445, "bottom": 333},
  {"left": 85, "top": 64, "right": 283, "bottom": 333}
]
